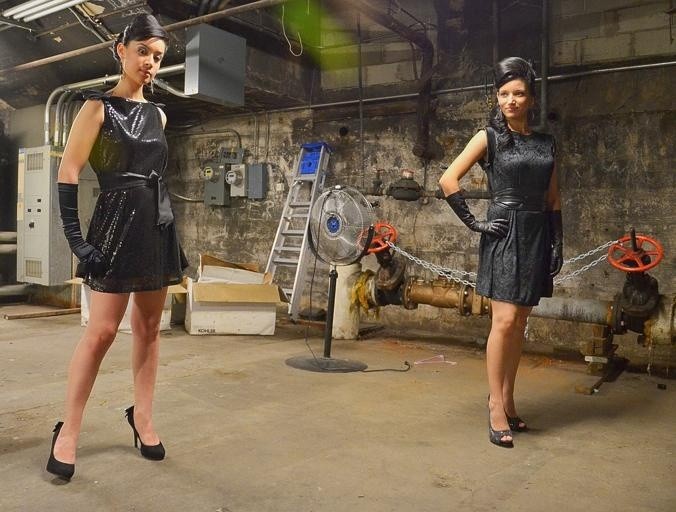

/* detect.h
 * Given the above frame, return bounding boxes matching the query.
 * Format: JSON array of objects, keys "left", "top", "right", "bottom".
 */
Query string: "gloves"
[
  {"left": 445, "top": 190, "right": 510, "bottom": 239},
  {"left": 548, "top": 210, "right": 563, "bottom": 279},
  {"left": 57, "top": 183, "right": 106, "bottom": 274}
]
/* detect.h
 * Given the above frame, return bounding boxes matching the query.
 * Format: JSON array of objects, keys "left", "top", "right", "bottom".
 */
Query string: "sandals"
[
  {"left": 504, "top": 407, "right": 526, "bottom": 432},
  {"left": 488, "top": 394, "right": 513, "bottom": 447}
]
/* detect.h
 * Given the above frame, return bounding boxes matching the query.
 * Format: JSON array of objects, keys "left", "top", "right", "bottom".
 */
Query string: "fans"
[{"left": 285, "top": 185, "right": 375, "bottom": 374}]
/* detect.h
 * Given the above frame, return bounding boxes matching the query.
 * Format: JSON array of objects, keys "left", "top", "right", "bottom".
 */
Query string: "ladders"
[{"left": 261, "top": 141, "right": 334, "bottom": 328}]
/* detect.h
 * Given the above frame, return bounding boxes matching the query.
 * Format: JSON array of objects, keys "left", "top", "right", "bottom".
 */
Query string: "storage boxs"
[{"left": 64, "top": 252, "right": 280, "bottom": 337}]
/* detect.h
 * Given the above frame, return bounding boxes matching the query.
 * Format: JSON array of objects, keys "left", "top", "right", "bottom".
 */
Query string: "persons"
[
  {"left": 435, "top": 56, "right": 567, "bottom": 452},
  {"left": 42, "top": 12, "right": 191, "bottom": 482}
]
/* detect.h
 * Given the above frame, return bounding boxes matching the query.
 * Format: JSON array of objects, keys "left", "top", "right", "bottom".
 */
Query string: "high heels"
[
  {"left": 47, "top": 422, "right": 74, "bottom": 480},
  {"left": 125, "top": 406, "right": 165, "bottom": 460}
]
